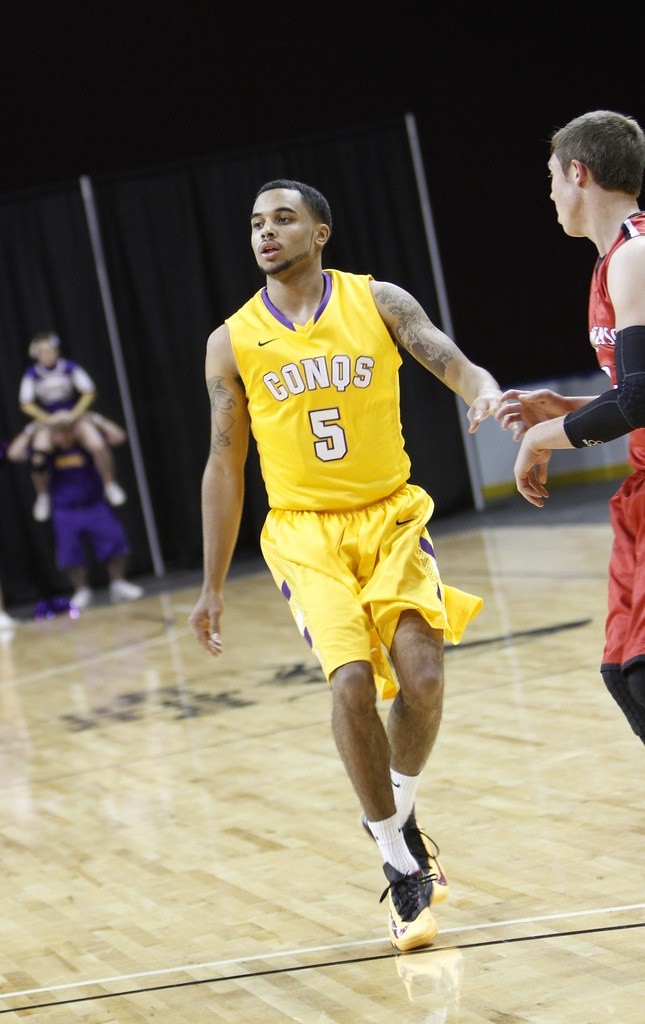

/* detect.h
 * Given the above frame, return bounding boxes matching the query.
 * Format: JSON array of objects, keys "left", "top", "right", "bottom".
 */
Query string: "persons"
[
  {"left": 8, "top": 332, "right": 141, "bottom": 608},
  {"left": 494, "top": 109, "right": 645, "bottom": 740},
  {"left": 188, "top": 181, "right": 520, "bottom": 952}
]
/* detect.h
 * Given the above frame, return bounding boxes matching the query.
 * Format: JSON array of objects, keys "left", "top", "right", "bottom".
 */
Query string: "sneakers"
[
  {"left": 104, "top": 482, "right": 126, "bottom": 505},
  {"left": 362, "top": 803, "right": 450, "bottom": 905},
  {"left": 383, "top": 864, "right": 437, "bottom": 950},
  {"left": 108, "top": 580, "right": 144, "bottom": 603},
  {"left": 34, "top": 492, "right": 51, "bottom": 522},
  {"left": 395, "top": 938, "right": 464, "bottom": 1019},
  {"left": 69, "top": 582, "right": 93, "bottom": 609}
]
